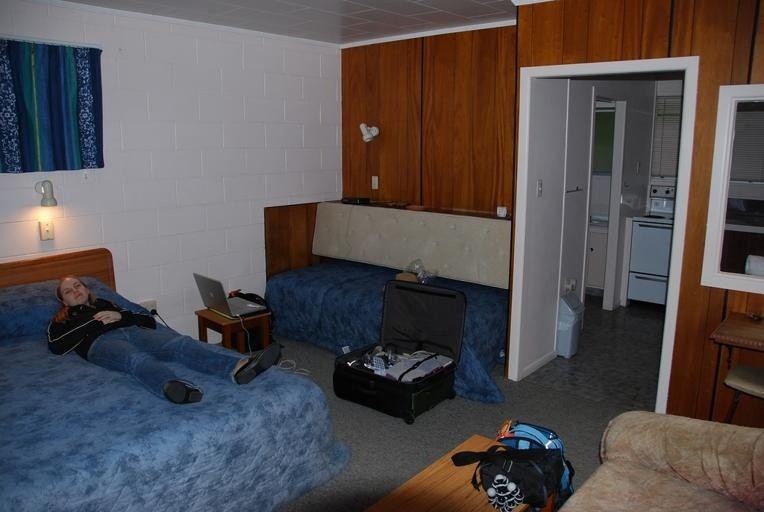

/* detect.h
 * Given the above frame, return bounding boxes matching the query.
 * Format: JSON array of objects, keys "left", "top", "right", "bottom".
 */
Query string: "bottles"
[{"left": 404, "top": 260, "right": 429, "bottom": 285}]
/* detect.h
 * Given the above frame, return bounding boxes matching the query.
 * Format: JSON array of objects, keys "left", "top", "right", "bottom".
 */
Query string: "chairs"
[{"left": 552, "top": 407, "right": 764, "bottom": 511}]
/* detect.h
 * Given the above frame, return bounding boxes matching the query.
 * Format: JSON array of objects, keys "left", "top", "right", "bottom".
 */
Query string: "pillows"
[{"left": 3, "top": 278, "right": 150, "bottom": 337}]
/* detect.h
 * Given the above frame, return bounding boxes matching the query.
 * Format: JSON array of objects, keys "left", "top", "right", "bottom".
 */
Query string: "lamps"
[
  {"left": 31, "top": 176, "right": 60, "bottom": 213},
  {"left": 354, "top": 116, "right": 379, "bottom": 146}
]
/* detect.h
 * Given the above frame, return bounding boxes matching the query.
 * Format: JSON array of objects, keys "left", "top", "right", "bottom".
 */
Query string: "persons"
[{"left": 45, "top": 275, "right": 281, "bottom": 407}]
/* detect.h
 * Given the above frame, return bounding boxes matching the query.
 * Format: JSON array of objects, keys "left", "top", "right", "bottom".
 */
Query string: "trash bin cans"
[{"left": 556, "top": 292, "right": 585, "bottom": 359}]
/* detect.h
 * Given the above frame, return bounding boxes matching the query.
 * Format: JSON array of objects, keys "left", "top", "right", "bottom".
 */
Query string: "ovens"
[{"left": 625, "top": 221, "right": 672, "bottom": 303}]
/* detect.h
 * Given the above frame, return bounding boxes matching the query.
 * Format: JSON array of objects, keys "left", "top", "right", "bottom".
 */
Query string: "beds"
[
  {"left": 264, "top": 198, "right": 510, "bottom": 376},
  {"left": 1, "top": 245, "right": 333, "bottom": 512}
]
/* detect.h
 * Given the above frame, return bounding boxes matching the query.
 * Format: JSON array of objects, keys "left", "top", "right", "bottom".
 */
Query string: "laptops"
[{"left": 193, "top": 273, "right": 267, "bottom": 318}]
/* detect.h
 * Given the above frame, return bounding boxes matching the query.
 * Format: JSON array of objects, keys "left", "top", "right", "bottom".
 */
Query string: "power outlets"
[{"left": 140, "top": 300, "right": 157, "bottom": 318}]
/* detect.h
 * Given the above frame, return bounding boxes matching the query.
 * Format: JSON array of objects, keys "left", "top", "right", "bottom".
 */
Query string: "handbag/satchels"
[
  {"left": 230, "top": 288, "right": 276, "bottom": 331},
  {"left": 451, "top": 415, "right": 575, "bottom": 507}
]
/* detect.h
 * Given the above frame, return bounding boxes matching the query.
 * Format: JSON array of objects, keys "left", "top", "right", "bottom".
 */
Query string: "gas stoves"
[{"left": 629, "top": 210, "right": 673, "bottom": 224}]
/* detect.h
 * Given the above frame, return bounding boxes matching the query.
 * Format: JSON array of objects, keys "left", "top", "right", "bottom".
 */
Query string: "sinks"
[{"left": 591, "top": 218, "right": 607, "bottom": 226}]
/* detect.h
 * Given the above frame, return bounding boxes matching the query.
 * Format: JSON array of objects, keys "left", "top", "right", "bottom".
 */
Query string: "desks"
[
  {"left": 709, "top": 309, "right": 762, "bottom": 426},
  {"left": 356, "top": 432, "right": 564, "bottom": 509}
]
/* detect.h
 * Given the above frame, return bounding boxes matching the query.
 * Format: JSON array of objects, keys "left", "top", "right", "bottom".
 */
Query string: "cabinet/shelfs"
[
  {"left": 584, "top": 231, "right": 607, "bottom": 289},
  {"left": 338, "top": 22, "right": 513, "bottom": 225}
]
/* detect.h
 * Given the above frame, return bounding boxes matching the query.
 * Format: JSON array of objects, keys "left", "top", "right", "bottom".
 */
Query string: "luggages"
[{"left": 332, "top": 279, "right": 467, "bottom": 425}]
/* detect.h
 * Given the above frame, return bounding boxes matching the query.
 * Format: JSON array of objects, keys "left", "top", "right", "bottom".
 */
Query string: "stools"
[
  {"left": 722, "top": 362, "right": 763, "bottom": 424},
  {"left": 193, "top": 299, "right": 274, "bottom": 354}
]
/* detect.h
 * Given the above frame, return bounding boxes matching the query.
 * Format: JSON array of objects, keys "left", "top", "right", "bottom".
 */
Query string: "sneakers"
[
  {"left": 233, "top": 342, "right": 281, "bottom": 385},
  {"left": 162, "top": 380, "right": 203, "bottom": 404}
]
